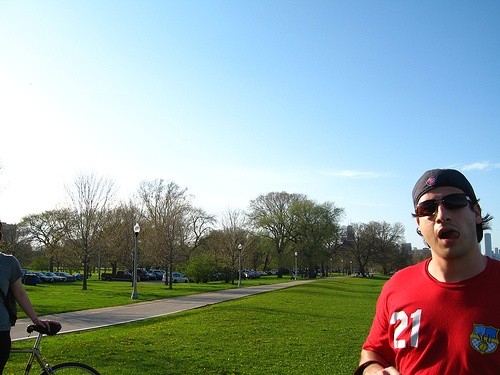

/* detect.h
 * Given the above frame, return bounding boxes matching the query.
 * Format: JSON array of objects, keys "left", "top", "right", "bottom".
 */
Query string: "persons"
[
  {"left": 75, "top": 272, "right": 81, "bottom": 277},
  {"left": 359, "top": 168, "right": 500, "bottom": 375},
  {"left": 0, "top": 222, "right": 52, "bottom": 375},
  {"left": 291, "top": 272, "right": 295, "bottom": 280}
]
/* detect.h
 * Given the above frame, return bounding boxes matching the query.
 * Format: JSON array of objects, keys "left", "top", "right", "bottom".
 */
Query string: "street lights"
[
  {"left": 238, "top": 243, "right": 243, "bottom": 286},
  {"left": 330, "top": 258, "right": 333, "bottom": 276},
  {"left": 350, "top": 261, "right": 352, "bottom": 274},
  {"left": 294, "top": 251, "right": 298, "bottom": 280},
  {"left": 341, "top": 260, "right": 343, "bottom": 276},
  {"left": 130, "top": 222, "right": 141, "bottom": 299}
]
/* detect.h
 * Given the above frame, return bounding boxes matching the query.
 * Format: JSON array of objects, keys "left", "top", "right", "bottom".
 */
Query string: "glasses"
[{"left": 413, "top": 193, "right": 472, "bottom": 217}]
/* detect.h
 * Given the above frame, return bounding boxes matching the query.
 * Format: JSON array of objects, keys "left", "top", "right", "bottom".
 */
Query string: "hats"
[{"left": 412, "top": 168, "right": 484, "bottom": 244}]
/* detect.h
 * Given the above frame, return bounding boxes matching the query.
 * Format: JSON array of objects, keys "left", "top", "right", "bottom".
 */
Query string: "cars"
[
  {"left": 241, "top": 268, "right": 289, "bottom": 280},
  {"left": 101, "top": 268, "right": 166, "bottom": 282},
  {"left": 21, "top": 269, "right": 85, "bottom": 285},
  {"left": 162, "top": 272, "right": 189, "bottom": 283},
  {"left": 293, "top": 268, "right": 309, "bottom": 275}
]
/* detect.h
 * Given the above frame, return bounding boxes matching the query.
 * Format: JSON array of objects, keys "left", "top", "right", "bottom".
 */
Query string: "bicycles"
[{"left": 8, "top": 320, "right": 101, "bottom": 375}]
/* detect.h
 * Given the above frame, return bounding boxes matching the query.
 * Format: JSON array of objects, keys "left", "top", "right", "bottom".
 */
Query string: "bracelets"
[{"left": 33, "top": 318, "right": 40, "bottom": 325}]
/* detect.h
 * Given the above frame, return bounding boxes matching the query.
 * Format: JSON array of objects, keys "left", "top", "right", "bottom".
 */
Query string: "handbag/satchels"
[{"left": 4, "top": 284, "right": 18, "bottom": 326}]
